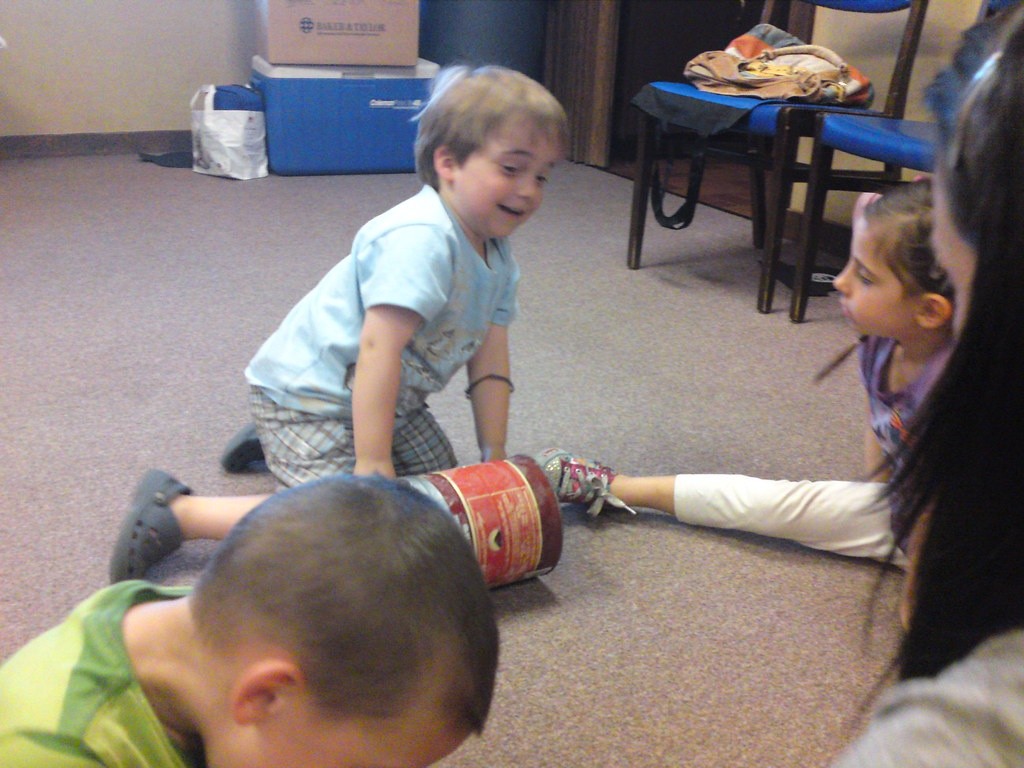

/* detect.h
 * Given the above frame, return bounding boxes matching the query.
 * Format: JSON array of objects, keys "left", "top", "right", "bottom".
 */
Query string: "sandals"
[
  {"left": 110, "top": 469, "right": 191, "bottom": 583},
  {"left": 222, "top": 424, "right": 264, "bottom": 472}
]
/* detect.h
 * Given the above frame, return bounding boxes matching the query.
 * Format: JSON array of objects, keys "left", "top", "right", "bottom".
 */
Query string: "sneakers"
[{"left": 534, "top": 446, "right": 637, "bottom": 517}]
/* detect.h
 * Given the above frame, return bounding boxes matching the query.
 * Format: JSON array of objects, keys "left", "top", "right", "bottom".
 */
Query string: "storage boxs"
[
  {"left": 268, "top": 0, "right": 419, "bottom": 66},
  {"left": 250, "top": 54, "right": 442, "bottom": 176}
]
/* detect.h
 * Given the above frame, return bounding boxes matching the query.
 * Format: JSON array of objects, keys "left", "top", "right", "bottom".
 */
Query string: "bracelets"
[{"left": 464, "top": 373, "right": 515, "bottom": 400}]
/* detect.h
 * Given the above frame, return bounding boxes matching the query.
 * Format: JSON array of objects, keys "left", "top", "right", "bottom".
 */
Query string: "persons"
[
  {"left": 0, "top": 473, "right": 501, "bottom": 768},
  {"left": 108, "top": 64, "right": 571, "bottom": 585},
  {"left": 830, "top": 18, "right": 1024, "bottom": 768},
  {"left": 534, "top": 178, "right": 956, "bottom": 574}
]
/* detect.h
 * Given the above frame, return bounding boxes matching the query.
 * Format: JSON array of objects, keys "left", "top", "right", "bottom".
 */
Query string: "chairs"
[
  {"left": 789, "top": 0, "right": 1024, "bottom": 322},
  {"left": 627, "top": 0, "right": 927, "bottom": 313}
]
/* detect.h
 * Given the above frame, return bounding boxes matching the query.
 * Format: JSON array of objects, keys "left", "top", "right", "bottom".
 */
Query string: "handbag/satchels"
[
  {"left": 188, "top": 83, "right": 269, "bottom": 181},
  {"left": 630, "top": 22, "right": 874, "bottom": 231}
]
